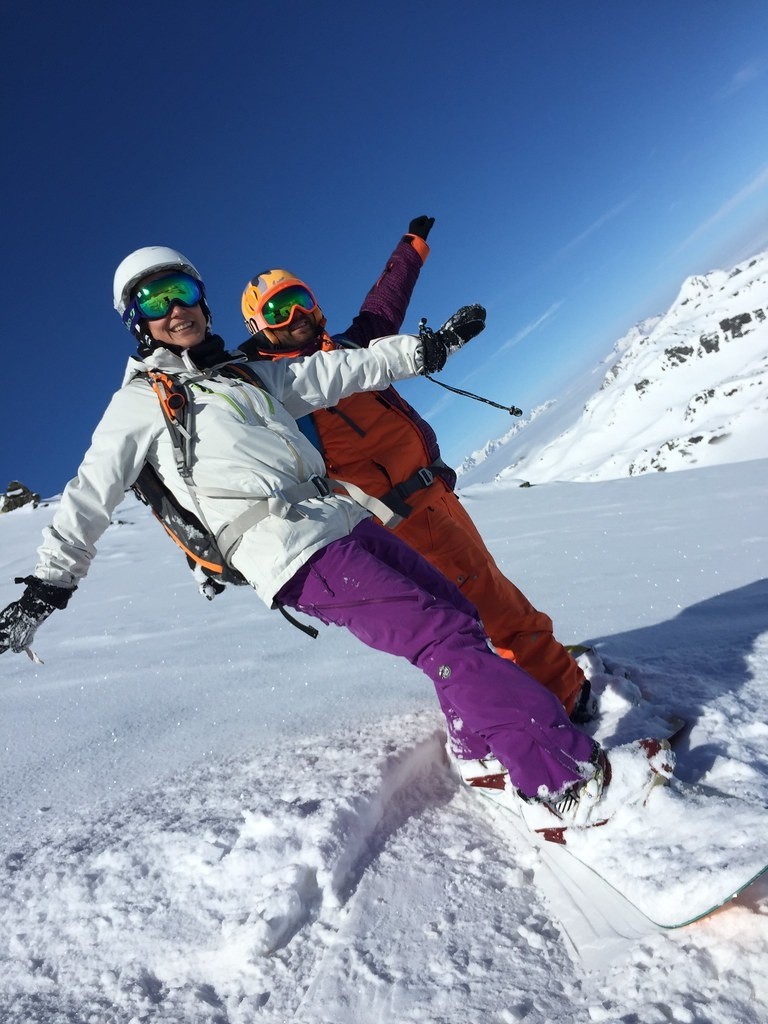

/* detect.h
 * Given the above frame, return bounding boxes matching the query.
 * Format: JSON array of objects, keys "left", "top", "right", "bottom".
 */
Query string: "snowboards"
[{"left": 423, "top": 646, "right": 767, "bottom": 928}]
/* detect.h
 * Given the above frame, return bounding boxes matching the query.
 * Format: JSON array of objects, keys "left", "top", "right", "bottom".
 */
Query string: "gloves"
[
  {"left": 409, "top": 214, "right": 436, "bottom": 242},
  {"left": 418, "top": 302, "right": 485, "bottom": 374},
  {"left": 0, "top": 576, "right": 74, "bottom": 655}
]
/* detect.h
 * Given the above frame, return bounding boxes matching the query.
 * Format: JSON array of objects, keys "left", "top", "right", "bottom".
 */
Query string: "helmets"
[
  {"left": 113, "top": 246, "right": 204, "bottom": 317},
  {"left": 241, "top": 272, "right": 322, "bottom": 345}
]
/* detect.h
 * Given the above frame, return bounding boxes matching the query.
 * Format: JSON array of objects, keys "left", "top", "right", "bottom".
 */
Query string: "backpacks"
[{"left": 130, "top": 354, "right": 270, "bottom": 600}]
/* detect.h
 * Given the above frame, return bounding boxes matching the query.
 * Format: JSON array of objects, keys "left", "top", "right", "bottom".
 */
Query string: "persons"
[
  {"left": 0, "top": 245, "right": 675, "bottom": 832},
  {"left": 242, "top": 216, "right": 644, "bottom": 727}
]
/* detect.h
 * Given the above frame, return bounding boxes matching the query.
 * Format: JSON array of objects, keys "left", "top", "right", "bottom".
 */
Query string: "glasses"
[
  {"left": 258, "top": 280, "right": 317, "bottom": 329},
  {"left": 135, "top": 273, "right": 202, "bottom": 321}
]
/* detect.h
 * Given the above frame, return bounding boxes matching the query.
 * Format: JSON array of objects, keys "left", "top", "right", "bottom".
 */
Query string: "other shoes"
[
  {"left": 458, "top": 759, "right": 511, "bottom": 792},
  {"left": 552, "top": 739, "right": 673, "bottom": 829}
]
